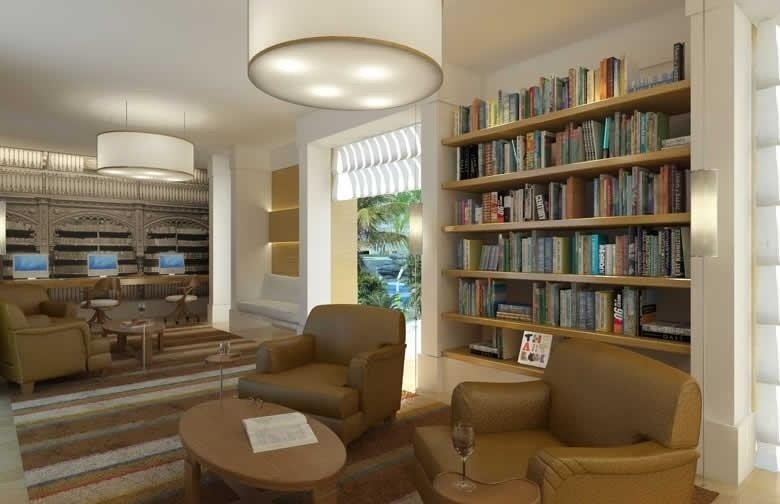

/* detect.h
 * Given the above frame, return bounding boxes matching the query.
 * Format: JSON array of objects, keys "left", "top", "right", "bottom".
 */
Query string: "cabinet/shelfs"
[{"left": 439, "top": 73, "right": 690, "bottom": 378}]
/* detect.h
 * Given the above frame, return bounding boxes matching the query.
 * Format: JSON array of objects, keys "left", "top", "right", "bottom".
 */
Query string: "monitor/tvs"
[
  {"left": 12, "top": 254, "right": 48, "bottom": 280},
  {"left": 87, "top": 254, "right": 118, "bottom": 278},
  {"left": 159, "top": 253, "right": 185, "bottom": 276}
]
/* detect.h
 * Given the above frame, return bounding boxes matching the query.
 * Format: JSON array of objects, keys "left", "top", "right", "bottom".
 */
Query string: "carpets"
[{"left": 10, "top": 323, "right": 720, "bottom": 503}]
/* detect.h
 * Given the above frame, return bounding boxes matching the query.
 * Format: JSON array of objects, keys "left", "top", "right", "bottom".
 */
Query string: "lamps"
[
  {"left": 245, "top": 0, "right": 446, "bottom": 114},
  {"left": 94, "top": 97, "right": 196, "bottom": 184}
]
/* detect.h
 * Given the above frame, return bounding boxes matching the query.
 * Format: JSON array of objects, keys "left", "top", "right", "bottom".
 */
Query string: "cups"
[
  {"left": 216, "top": 341, "right": 231, "bottom": 359},
  {"left": 137, "top": 302, "right": 147, "bottom": 320}
]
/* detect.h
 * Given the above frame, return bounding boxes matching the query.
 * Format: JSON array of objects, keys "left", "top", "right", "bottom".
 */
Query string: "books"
[
  {"left": 122, "top": 319, "right": 152, "bottom": 328},
  {"left": 242, "top": 411, "right": 318, "bottom": 453}
]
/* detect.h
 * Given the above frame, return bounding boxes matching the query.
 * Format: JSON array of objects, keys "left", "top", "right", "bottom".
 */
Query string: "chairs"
[
  {"left": 0, "top": 272, "right": 201, "bottom": 400},
  {"left": 412, "top": 337, "right": 703, "bottom": 504},
  {"left": 236, "top": 303, "right": 407, "bottom": 450}
]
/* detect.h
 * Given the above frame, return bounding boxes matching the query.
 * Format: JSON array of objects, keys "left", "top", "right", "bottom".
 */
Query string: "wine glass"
[{"left": 450, "top": 422, "right": 478, "bottom": 492}]
